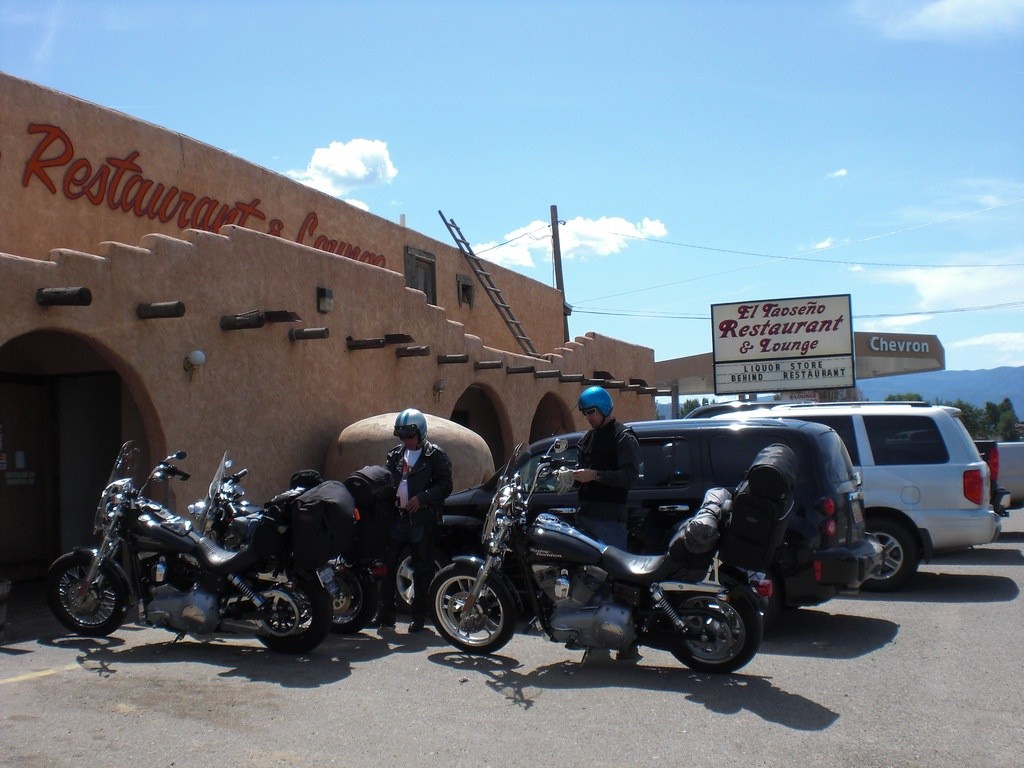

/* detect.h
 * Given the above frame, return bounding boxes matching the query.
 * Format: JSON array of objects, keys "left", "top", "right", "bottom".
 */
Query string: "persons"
[
  {"left": 558, "top": 387, "right": 642, "bottom": 659},
  {"left": 369, "top": 409, "right": 453, "bottom": 631}
]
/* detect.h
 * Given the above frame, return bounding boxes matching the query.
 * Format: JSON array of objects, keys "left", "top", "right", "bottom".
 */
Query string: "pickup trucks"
[{"left": 996, "top": 442, "right": 1024, "bottom": 509}]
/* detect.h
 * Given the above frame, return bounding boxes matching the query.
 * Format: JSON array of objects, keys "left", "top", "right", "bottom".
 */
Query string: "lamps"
[
  {"left": 184, "top": 350, "right": 205, "bottom": 371},
  {"left": 317, "top": 286, "right": 334, "bottom": 313}
]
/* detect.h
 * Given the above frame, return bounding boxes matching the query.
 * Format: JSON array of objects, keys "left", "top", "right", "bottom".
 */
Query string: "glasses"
[
  {"left": 582, "top": 408, "right": 598, "bottom": 416},
  {"left": 400, "top": 432, "right": 419, "bottom": 440}
]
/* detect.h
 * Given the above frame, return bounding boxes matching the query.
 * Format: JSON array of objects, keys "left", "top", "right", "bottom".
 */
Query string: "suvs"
[
  {"left": 381, "top": 417, "right": 886, "bottom": 634},
  {"left": 712, "top": 401, "right": 1004, "bottom": 590},
  {"left": 669, "top": 398, "right": 1012, "bottom": 520}
]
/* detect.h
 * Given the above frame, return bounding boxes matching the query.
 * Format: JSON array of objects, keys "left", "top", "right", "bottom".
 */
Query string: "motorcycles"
[
  {"left": 188, "top": 446, "right": 388, "bottom": 635},
  {"left": 42, "top": 439, "right": 353, "bottom": 656},
  {"left": 425, "top": 437, "right": 796, "bottom": 673}
]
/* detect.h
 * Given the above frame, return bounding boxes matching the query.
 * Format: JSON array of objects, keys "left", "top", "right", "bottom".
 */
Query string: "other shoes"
[
  {"left": 408, "top": 616, "right": 425, "bottom": 633},
  {"left": 364, "top": 616, "right": 395, "bottom": 628},
  {"left": 619, "top": 647, "right": 638, "bottom": 658}
]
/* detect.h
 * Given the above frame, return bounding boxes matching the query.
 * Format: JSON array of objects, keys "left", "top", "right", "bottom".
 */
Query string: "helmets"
[
  {"left": 394, "top": 408, "right": 427, "bottom": 440},
  {"left": 578, "top": 386, "right": 614, "bottom": 417}
]
